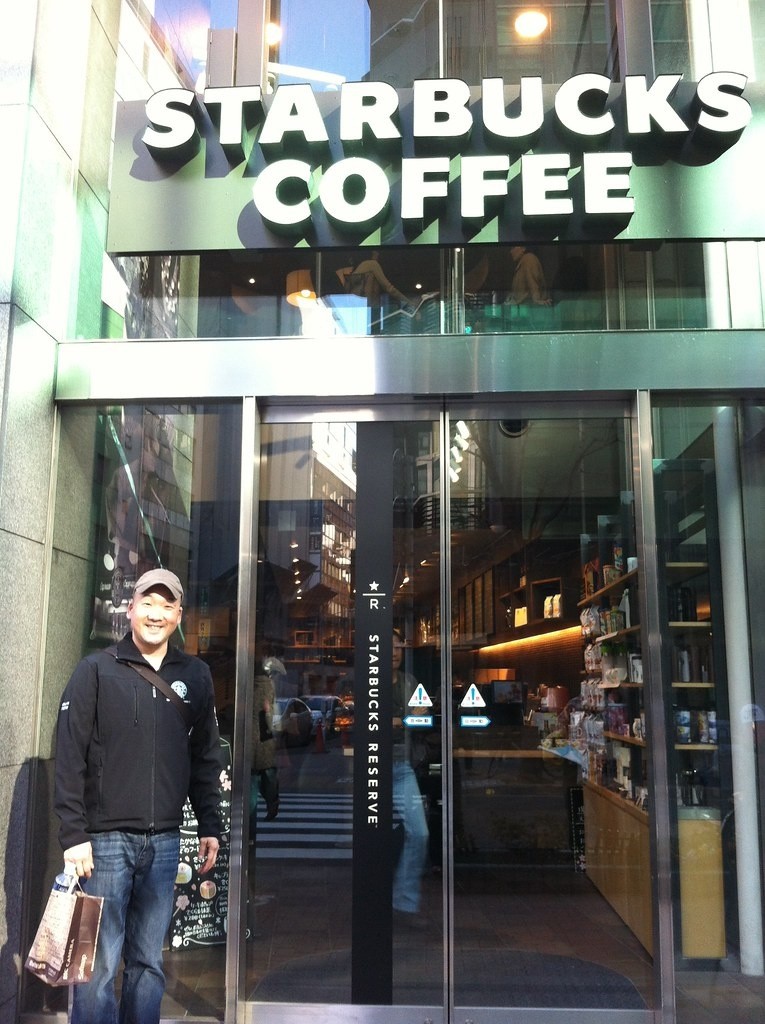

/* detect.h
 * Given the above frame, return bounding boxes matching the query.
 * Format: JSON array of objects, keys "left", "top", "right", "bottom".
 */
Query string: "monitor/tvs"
[{"left": 490, "top": 679, "right": 524, "bottom": 705}]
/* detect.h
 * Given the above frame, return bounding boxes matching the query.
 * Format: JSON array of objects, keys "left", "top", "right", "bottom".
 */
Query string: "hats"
[{"left": 133, "top": 568, "right": 185, "bottom": 605}]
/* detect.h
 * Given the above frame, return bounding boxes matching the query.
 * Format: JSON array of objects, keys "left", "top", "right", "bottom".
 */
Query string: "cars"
[
  {"left": 272, "top": 697, "right": 314, "bottom": 747},
  {"left": 297, "top": 695, "right": 355, "bottom": 742}
]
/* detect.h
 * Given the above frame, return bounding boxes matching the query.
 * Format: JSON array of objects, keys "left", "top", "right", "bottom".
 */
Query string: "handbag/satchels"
[
  {"left": 258, "top": 703, "right": 284, "bottom": 742},
  {"left": 24, "top": 871, "right": 104, "bottom": 987}
]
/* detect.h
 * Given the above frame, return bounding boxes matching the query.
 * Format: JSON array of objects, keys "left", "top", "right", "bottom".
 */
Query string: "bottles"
[
  {"left": 51, "top": 864, "right": 76, "bottom": 895},
  {"left": 598, "top": 605, "right": 618, "bottom": 635}
]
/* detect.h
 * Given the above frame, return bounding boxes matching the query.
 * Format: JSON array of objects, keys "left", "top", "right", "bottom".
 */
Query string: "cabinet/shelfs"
[
  {"left": 581, "top": 558, "right": 732, "bottom": 965},
  {"left": 496, "top": 577, "right": 567, "bottom": 634}
]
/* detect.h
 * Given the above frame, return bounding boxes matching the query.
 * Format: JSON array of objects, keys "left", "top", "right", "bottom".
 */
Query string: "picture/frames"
[{"left": 456, "top": 567, "right": 496, "bottom": 644}]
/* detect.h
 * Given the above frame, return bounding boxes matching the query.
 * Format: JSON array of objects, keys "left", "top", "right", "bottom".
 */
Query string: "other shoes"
[
  {"left": 264, "top": 798, "right": 282, "bottom": 820},
  {"left": 391, "top": 912, "right": 436, "bottom": 931}
]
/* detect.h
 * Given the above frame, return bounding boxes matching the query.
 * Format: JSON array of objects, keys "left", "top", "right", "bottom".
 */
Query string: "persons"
[
  {"left": 55, "top": 568, "right": 221, "bottom": 1024},
  {"left": 392, "top": 630, "right": 429, "bottom": 929},
  {"left": 498, "top": 684, "right": 520, "bottom": 703},
  {"left": 187, "top": 585, "right": 281, "bottom": 937}
]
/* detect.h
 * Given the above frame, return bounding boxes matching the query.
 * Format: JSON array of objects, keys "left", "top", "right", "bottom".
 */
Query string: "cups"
[
  {"left": 603, "top": 564, "right": 615, "bottom": 587},
  {"left": 627, "top": 557, "right": 638, "bottom": 572},
  {"left": 627, "top": 647, "right": 644, "bottom": 683},
  {"left": 608, "top": 703, "right": 718, "bottom": 745}
]
